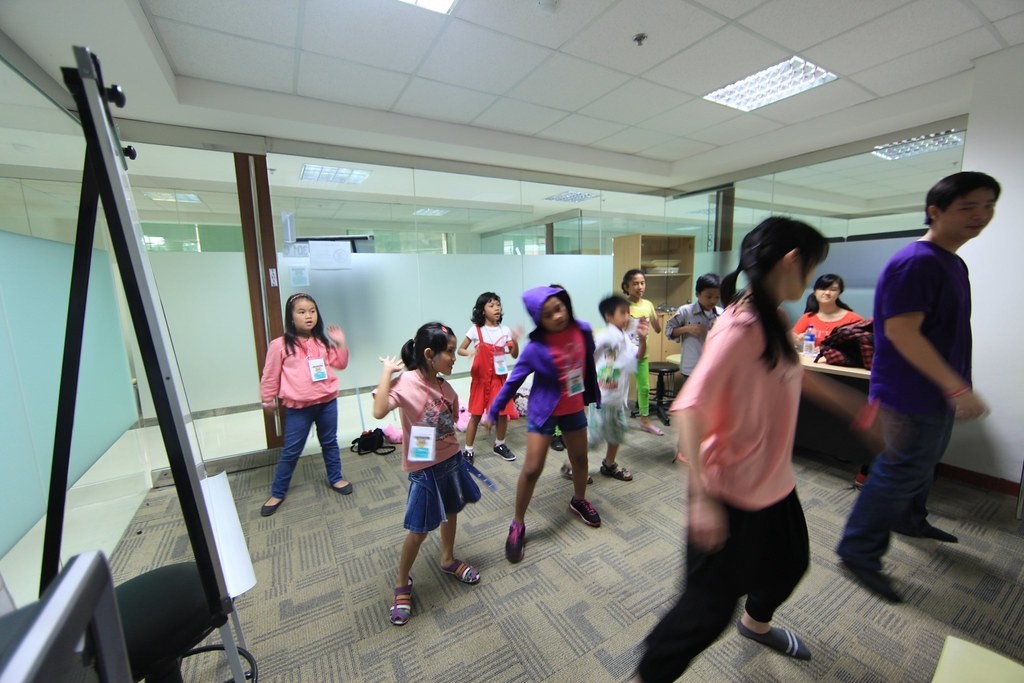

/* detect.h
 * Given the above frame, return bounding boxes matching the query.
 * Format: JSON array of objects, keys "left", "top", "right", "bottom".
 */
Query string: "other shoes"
[
  {"left": 853, "top": 464, "right": 868, "bottom": 487},
  {"left": 331, "top": 480, "right": 353, "bottom": 495},
  {"left": 261, "top": 496, "right": 284, "bottom": 516}
]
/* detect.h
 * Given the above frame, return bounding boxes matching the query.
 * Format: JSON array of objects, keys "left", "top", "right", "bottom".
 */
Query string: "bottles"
[{"left": 803, "top": 325, "right": 815, "bottom": 358}]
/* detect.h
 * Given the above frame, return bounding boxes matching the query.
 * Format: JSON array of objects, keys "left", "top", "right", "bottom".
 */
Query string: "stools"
[
  {"left": 631, "top": 361, "right": 680, "bottom": 425},
  {"left": 665, "top": 354, "right": 682, "bottom": 397}
]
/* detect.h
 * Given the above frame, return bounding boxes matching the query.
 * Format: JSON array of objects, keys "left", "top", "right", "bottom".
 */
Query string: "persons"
[
  {"left": 559, "top": 295, "right": 649, "bottom": 486},
  {"left": 620, "top": 215, "right": 830, "bottom": 683},
  {"left": 836, "top": 168, "right": 1002, "bottom": 604},
  {"left": 455, "top": 289, "right": 519, "bottom": 462},
  {"left": 662, "top": 272, "right": 725, "bottom": 465},
  {"left": 791, "top": 272, "right": 861, "bottom": 361},
  {"left": 620, "top": 269, "right": 664, "bottom": 436},
  {"left": 484, "top": 285, "right": 603, "bottom": 566},
  {"left": 371, "top": 321, "right": 481, "bottom": 625},
  {"left": 259, "top": 293, "right": 348, "bottom": 519}
]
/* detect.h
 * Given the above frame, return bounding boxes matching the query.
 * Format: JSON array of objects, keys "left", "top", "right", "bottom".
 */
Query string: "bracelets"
[
  {"left": 946, "top": 383, "right": 972, "bottom": 401},
  {"left": 261, "top": 401, "right": 274, "bottom": 407}
]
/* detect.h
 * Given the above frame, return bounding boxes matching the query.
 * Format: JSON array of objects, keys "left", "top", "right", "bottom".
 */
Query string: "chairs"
[{"left": 0, "top": 547, "right": 258, "bottom": 683}]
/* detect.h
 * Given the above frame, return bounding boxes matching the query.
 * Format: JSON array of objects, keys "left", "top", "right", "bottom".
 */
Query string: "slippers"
[{"left": 640, "top": 423, "right": 664, "bottom": 436}]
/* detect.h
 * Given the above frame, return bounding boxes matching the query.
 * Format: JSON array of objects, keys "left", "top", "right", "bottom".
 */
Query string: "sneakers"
[
  {"left": 570, "top": 496, "right": 601, "bottom": 527},
  {"left": 558, "top": 433, "right": 567, "bottom": 447},
  {"left": 492, "top": 444, "right": 516, "bottom": 461},
  {"left": 505, "top": 520, "right": 525, "bottom": 563},
  {"left": 463, "top": 450, "right": 474, "bottom": 467},
  {"left": 550, "top": 434, "right": 564, "bottom": 450}
]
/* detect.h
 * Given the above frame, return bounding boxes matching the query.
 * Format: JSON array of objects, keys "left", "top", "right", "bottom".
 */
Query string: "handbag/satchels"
[
  {"left": 350, "top": 428, "right": 395, "bottom": 457},
  {"left": 815, "top": 318, "right": 873, "bottom": 370}
]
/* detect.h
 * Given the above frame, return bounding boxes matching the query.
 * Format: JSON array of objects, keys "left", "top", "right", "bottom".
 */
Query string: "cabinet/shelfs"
[{"left": 612, "top": 233, "right": 696, "bottom": 398}]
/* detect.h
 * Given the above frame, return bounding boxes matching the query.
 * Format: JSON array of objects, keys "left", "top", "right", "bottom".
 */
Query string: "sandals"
[
  {"left": 441, "top": 559, "right": 480, "bottom": 583},
  {"left": 560, "top": 465, "right": 594, "bottom": 484},
  {"left": 600, "top": 459, "right": 633, "bottom": 481},
  {"left": 389, "top": 576, "right": 413, "bottom": 624}
]
